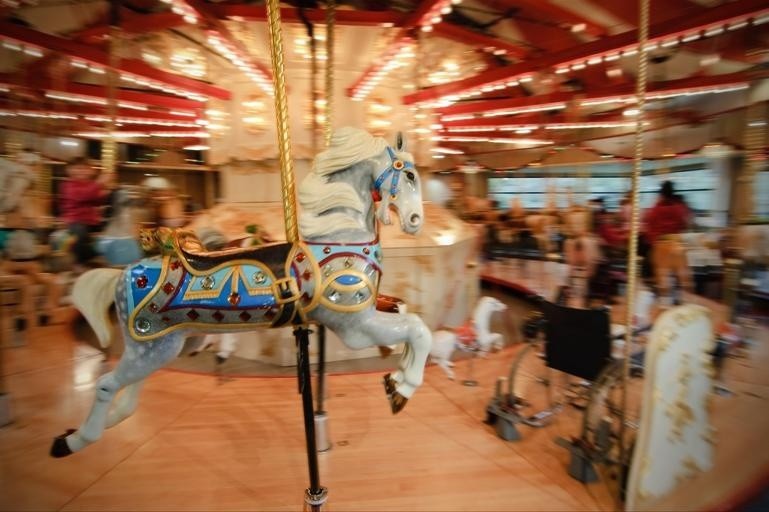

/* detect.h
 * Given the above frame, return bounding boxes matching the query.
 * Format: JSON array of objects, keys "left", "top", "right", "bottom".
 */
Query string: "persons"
[
  {"left": 454, "top": 185, "right": 643, "bottom": 266},
  {"left": 646, "top": 179, "right": 695, "bottom": 299},
  {"left": 60, "top": 155, "right": 108, "bottom": 267}
]
